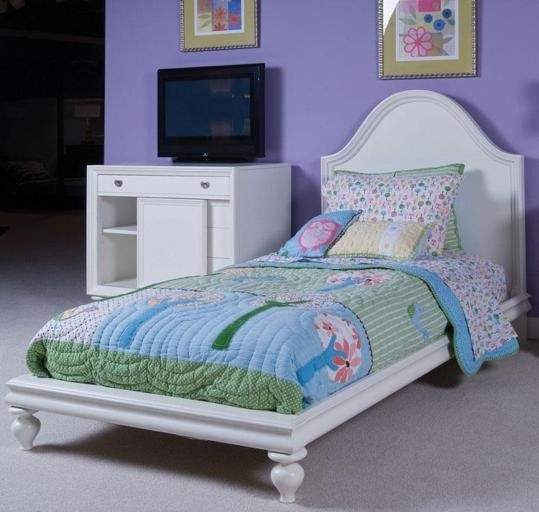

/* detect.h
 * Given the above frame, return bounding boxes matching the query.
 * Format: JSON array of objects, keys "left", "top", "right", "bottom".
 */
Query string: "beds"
[{"left": 3, "top": 85, "right": 535, "bottom": 505}]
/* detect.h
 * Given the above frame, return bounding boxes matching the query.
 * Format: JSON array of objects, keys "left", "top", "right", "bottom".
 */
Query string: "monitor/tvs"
[{"left": 155, "top": 62, "right": 267, "bottom": 165}]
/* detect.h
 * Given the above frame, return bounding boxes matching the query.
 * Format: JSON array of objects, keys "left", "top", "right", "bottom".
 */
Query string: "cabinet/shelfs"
[{"left": 79, "top": 158, "right": 296, "bottom": 304}]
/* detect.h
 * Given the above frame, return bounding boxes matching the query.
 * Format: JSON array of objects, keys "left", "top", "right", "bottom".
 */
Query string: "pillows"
[
  {"left": 324, "top": 172, "right": 462, "bottom": 255},
  {"left": 338, "top": 161, "right": 463, "bottom": 253},
  {"left": 282, "top": 207, "right": 363, "bottom": 261},
  {"left": 333, "top": 216, "right": 431, "bottom": 259}
]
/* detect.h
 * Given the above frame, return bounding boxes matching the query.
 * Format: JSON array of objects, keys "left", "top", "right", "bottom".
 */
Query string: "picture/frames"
[
  {"left": 375, "top": 2, "right": 481, "bottom": 84},
  {"left": 175, "top": 0, "right": 262, "bottom": 55}
]
